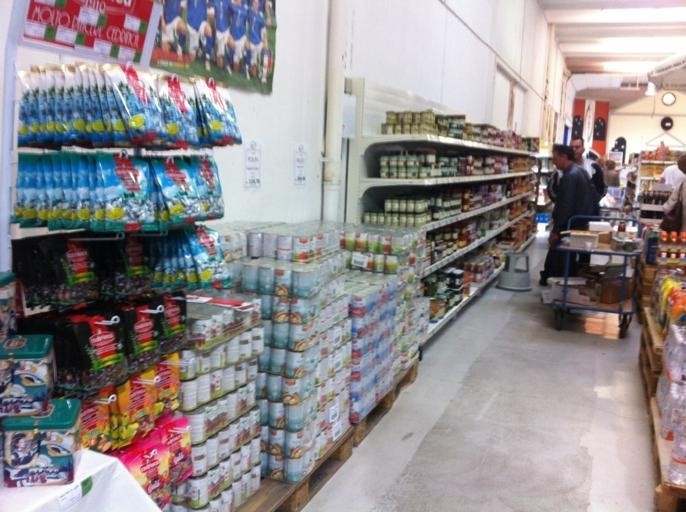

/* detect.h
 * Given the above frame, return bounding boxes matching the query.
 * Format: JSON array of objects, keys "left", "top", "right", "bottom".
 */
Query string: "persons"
[
  {"left": 539, "top": 136, "right": 619, "bottom": 286},
  {"left": 158, "top": 0, "right": 273, "bottom": 84},
  {"left": 662, "top": 155, "right": 686, "bottom": 233},
  {"left": 660, "top": 163, "right": 686, "bottom": 191}
]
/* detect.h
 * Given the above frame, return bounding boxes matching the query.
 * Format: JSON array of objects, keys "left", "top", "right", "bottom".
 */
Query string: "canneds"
[{"left": 163, "top": 109, "right": 465, "bottom": 512}]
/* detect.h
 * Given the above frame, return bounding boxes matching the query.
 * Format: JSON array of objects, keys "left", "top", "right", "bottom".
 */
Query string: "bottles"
[
  {"left": 640, "top": 190, "right": 674, "bottom": 218},
  {"left": 656, "top": 225, "right": 686, "bottom": 486},
  {"left": 618, "top": 221, "right": 626, "bottom": 232}
]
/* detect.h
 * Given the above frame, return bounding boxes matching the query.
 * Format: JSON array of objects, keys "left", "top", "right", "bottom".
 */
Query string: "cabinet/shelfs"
[
  {"left": 343, "top": 136, "right": 558, "bottom": 346},
  {"left": 623, "top": 148, "right": 686, "bottom": 233}
]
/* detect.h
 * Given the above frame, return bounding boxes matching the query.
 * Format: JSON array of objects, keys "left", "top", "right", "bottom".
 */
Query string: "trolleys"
[{"left": 549, "top": 216, "right": 642, "bottom": 340}]
